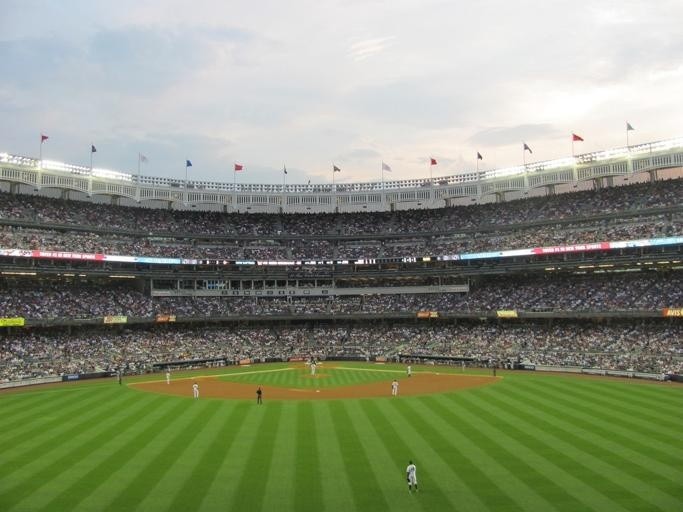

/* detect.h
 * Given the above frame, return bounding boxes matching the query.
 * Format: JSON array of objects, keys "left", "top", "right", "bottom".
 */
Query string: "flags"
[
  {"left": 41, "top": 134, "right": 48, "bottom": 144},
  {"left": 334, "top": 166, "right": 340, "bottom": 172},
  {"left": 572, "top": 134, "right": 583, "bottom": 142},
  {"left": 139, "top": 153, "right": 147, "bottom": 162},
  {"left": 524, "top": 143, "right": 532, "bottom": 153},
  {"left": 382, "top": 162, "right": 391, "bottom": 172},
  {"left": 627, "top": 122, "right": 634, "bottom": 131},
  {"left": 186, "top": 159, "right": 192, "bottom": 168},
  {"left": 91, "top": 145, "right": 96, "bottom": 153},
  {"left": 234, "top": 164, "right": 243, "bottom": 171},
  {"left": 284, "top": 165, "right": 287, "bottom": 174},
  {"left": 431, "top": 158, "right": 438, "bottom": 166},
  {"left": 477, "top": 153, "right": 483, "bottom": 161}
]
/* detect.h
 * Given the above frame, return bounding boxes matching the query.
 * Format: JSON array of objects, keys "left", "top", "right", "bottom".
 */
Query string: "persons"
[
  {"left": 391, "top": 380, "right": 398, "bottom": 396},
  {"left": 192, "top": 382, "right": 199, "bottom": 398},
  {"left": 0, "top": 185, "right": 683, "bottom": 379},
  {"left": 405, "top": 460, "right": 419, "bottom": 494},
  {"left": 116, "top": 373, "right": 122, "bottom": 384},
  {"left": 165, "top": 371, "right": 170, "bottom": 385},
  {"left": 255, "top": 385, "right": 261, "bottom": 404}
]
